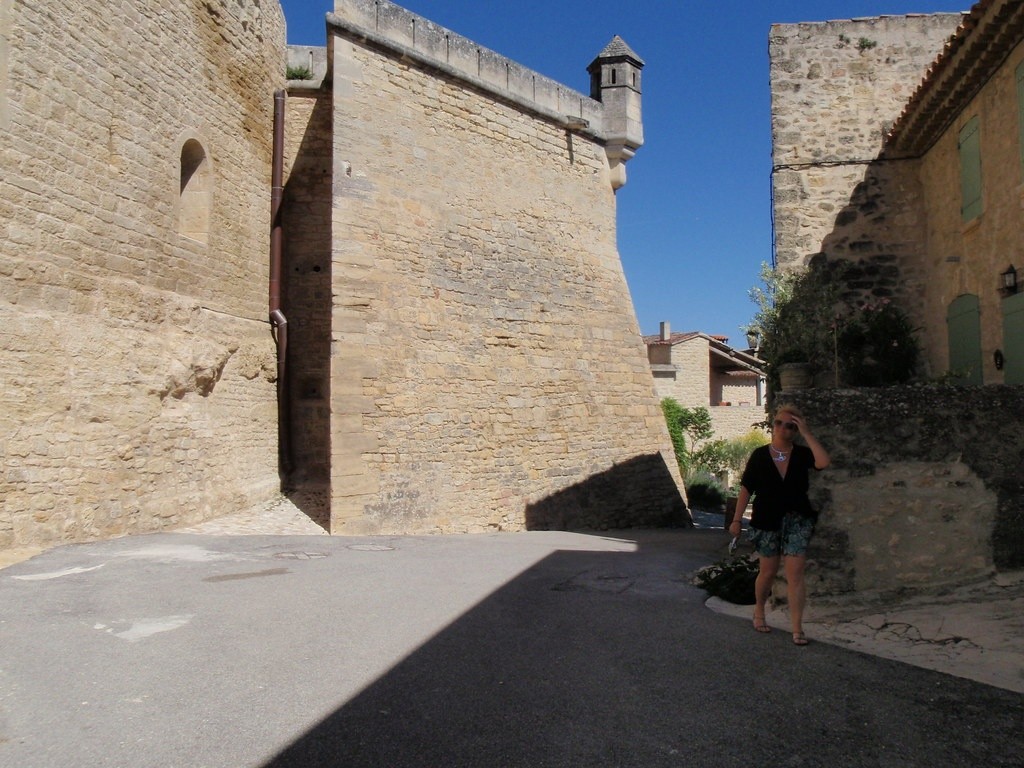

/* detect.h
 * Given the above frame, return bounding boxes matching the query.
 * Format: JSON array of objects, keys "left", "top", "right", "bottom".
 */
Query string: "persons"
[{"left": 729, "top": 404, "right": 831, "bottom": 647}]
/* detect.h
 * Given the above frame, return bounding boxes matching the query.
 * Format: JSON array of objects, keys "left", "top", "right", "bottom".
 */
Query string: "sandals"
[
  {"left": 752, "top": 614, "right": 771, "bottom": 632},
  {"left": 792, "top": 631, "right": 808, "bottom": 645}
]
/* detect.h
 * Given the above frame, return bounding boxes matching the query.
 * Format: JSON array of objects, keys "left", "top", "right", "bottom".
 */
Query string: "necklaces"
[{"left": 770, "top": 443, "right": 793, "bottom": 462}]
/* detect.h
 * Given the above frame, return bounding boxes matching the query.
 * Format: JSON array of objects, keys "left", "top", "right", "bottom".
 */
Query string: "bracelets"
[{"left": 730, "top": 519, "right": 743, "bottom": 525}]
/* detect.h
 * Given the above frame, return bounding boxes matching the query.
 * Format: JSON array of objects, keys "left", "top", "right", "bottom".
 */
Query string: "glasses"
[{"left": 772, "top": 419, "right": 797, "bottom": 432}]
